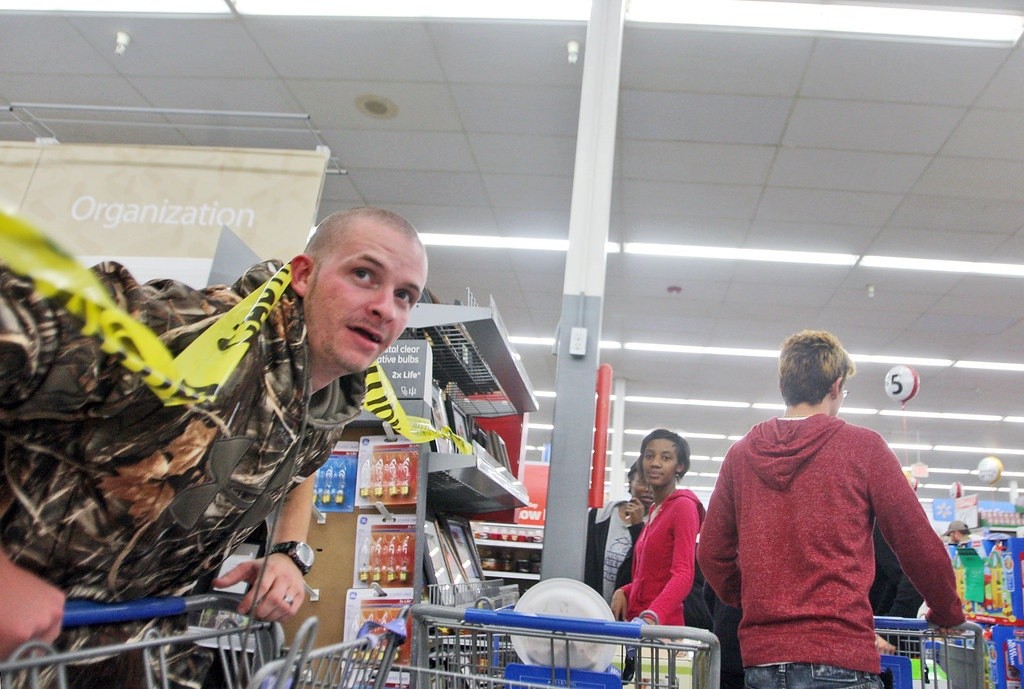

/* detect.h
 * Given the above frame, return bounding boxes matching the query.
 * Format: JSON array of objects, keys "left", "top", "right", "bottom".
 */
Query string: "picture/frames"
[
  {"left": 424, "top": 515, "right": 486, "bottom": 607},
  {"left": 430, "top": 381, "right": 512, "bottom": 474}
]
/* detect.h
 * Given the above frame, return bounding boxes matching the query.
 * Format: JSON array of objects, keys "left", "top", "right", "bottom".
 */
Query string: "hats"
[{"left": 941, "top": 520, "right": 971, "bottom": 538}]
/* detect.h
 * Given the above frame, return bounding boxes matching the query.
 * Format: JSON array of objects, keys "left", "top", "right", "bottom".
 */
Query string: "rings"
[{"left": 283, "top": 594, "right": 294, "bottom": 606}]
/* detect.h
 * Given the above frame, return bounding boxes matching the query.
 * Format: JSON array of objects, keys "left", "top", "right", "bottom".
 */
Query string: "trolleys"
[
  {"left": 411, "top": 596, "right": 721, "bottom": 689},
  {"left": 873, "top": 615, "right": 987, "bottom": 689},
  {"left": 1, "top": 591, "right": 409, "bottom": 689}
]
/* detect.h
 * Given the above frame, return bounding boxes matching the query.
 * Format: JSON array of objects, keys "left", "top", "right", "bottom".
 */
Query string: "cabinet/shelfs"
[
  {"left": 470, "top": 520, "right": 544, "bottom": 598},
  {"left": 273, "top": 287, "right": 540, "bottom": 689}
]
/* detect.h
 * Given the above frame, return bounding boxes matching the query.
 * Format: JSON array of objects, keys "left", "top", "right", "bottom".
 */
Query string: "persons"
[
  {"left": 0, "top": 207, "right": 429, "bottom": 689},
  {"left": 697, "top": 329, "right": 967, "bottom": 689},
  {"left": 586, "top": 429, "right": 706, "bottom": 626},
  {"left": 941, "top": 520, "right": 972, "bottom": 542}
]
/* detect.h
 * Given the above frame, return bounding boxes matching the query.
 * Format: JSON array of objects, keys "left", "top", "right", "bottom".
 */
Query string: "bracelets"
[{"left": 639, "top": 610, "right": 659, "bottom": 625}]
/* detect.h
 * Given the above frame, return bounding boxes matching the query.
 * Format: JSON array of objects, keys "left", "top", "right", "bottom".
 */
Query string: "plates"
[{"left": 509, "top": 577, "right": 616, "bottom": 673}]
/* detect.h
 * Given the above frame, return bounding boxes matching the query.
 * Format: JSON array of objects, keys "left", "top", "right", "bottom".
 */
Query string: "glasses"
[{"left": 841, "top": 389, "right": 849, "bottom": 398}]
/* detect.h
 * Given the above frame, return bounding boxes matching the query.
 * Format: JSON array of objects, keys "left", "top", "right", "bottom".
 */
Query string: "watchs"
[{"left": 270, "top": 540, "right": 315, "bottom": 577}]
